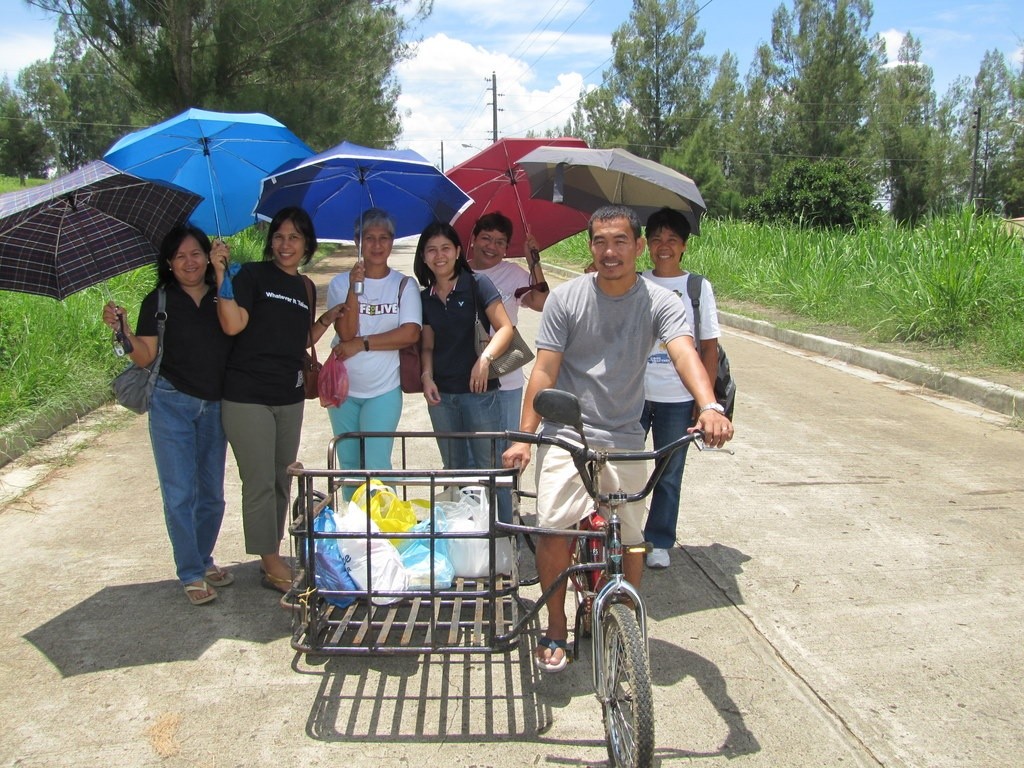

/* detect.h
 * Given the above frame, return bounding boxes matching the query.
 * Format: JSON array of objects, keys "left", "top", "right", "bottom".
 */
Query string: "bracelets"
[
  {"left": 363, "top": 336, "right": 369, "bottom": 351},
  {"left": 421, "top": 371, "right": 433, "bottom": 383},
  {"left": 528, "top": 262, "right": 539, "bottom": 269},
  {"left": 485, "top": 353, "right": 494, "bottom": 362},
  {"left": 318, "top": 318, "right": 328, "bottom": 327},
  {"left": 127, "top": 329, "right": 132, "bottom": 337}
]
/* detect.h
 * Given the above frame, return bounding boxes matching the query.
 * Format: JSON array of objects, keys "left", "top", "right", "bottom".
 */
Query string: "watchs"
[{"left": 700, "top": 402, "right": 725, "bottom": 415}]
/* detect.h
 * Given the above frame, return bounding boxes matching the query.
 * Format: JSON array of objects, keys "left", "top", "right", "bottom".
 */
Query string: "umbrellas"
[
  {"left": 250, "top": 141, "right": 477, "bottom": 297},
  {"left": 513, "top": 145, "right": 708, "bottom": 237},
  {"left": 446, "top": 138, "right": 592, "bottom": 262},
  {"left": 0, "top": 159, "right": 205, "bottom": 355},
  {"left": 103, "top": 109, "right": 315, "bottom": 300}
]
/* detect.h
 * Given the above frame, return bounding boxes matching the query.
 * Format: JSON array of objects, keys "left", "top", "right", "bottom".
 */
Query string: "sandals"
[
  {"left": 260, "top": 560, "right": 290, "bottom": 573},
  {"left": 262, "top": 573, "right": 296, "bottom": 598}
]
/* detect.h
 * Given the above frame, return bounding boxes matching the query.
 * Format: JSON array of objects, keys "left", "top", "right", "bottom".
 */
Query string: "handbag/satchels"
[
  {"left": 317, "top": 350, "right": 349, "bottom": 408},
  {"left": 301, "top": 350, "right": 322, "bottom": 399},
  {"left": 398, "top": 276, "right": 424, "bottom": 393},
  {"left": 707, "top": 343, "right": 736, "bottom": 423},
  {"left": 109, "top": 286, "right": 167, "bottom": 415},
  {"left": 469, "top": 273, "right": 535, "bottom": 379},
  {"left": 302, "top": 480, "right": 511, "bottom": 609}
]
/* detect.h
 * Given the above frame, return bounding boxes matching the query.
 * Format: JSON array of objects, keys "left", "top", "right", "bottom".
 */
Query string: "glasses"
[
  {"left": 362, "top": 234, "right": 392, "bottom": 244},
  {"left": 112, "top": 314, "right": 133, "bottom": 355},
  {"left": 477, "top": 234, "right": 507, "bottom": 249}
]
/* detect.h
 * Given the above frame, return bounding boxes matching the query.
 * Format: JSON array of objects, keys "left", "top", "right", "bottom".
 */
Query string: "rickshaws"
[{"left": 281, "top": 386, "right": 735, "bottom": 768}]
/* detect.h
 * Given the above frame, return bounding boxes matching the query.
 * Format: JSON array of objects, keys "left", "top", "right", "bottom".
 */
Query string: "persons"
[
  {"left": 463, "top": 210, "right": 549, "bottom": 468},
  {"left": 501, "top": 204, "right": 735, "bottom": 673},
  {"left": 413, "top": 221, "right": 514, "bottom": 524},
  {"left": 211, "top": 206, "right": 349, "bottom": 595},
  {"left": 584, "top": 206, "right": 721, "bottom": 569},
  {"left": 102, "top": 225, "right": 236, "bottom": 604},
  {"left": 326, "top": 208, "right": 422, "bottom": 503}
]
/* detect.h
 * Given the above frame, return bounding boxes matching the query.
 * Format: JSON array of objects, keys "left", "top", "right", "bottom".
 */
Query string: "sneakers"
[{"left": 646, "top": 548, "right": 670, "bottom": 567}]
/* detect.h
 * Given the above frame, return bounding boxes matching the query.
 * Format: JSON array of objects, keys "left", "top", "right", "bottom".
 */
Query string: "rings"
[
  {"left": 475, "top": 382, "right": 478, "bottom": 385},
  {"left": 722, "top": 428, "right": 727, "bottom": 430}
]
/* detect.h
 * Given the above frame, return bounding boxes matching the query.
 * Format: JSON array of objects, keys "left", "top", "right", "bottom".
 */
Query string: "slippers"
[
  {"left": 532, "top": 633, "right": 568, "bottom": 672},
  {"left": 204, "top": 565, "right": 234, "bottom": 587},
  {"left": 181, "top": 580, "right": 217, "bottom": 605}
]
